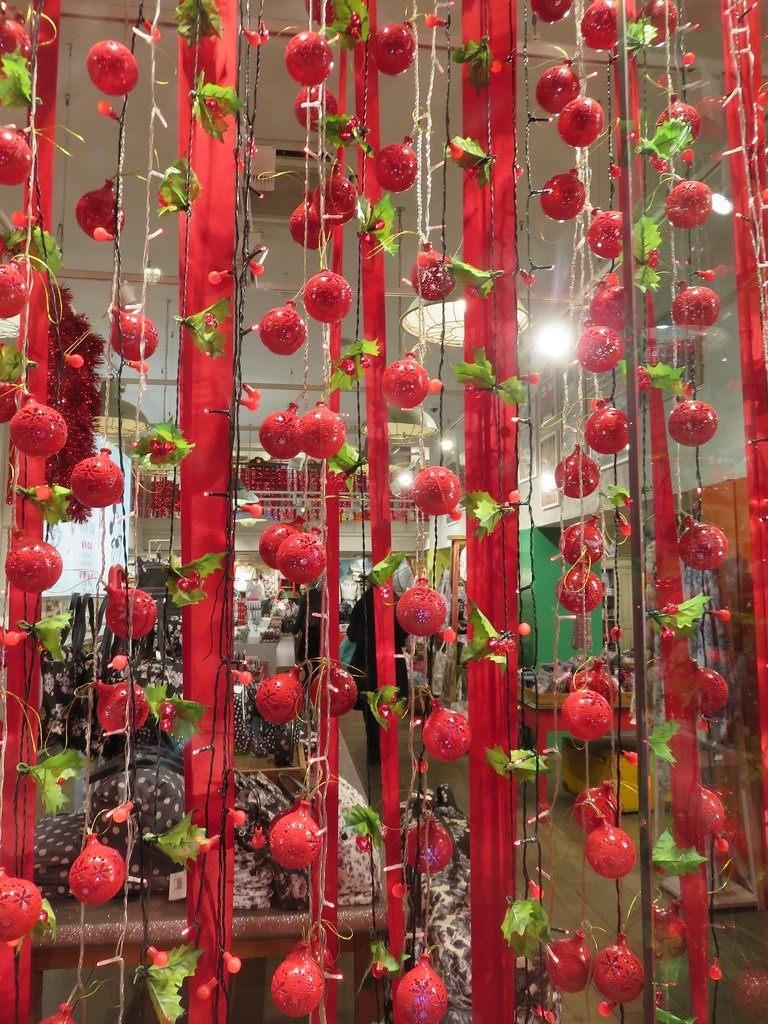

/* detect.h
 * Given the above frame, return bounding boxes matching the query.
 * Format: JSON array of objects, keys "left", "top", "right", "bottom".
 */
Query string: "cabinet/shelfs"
[{"left": 599, "top": 559, "right": 635, "bottom": 654}]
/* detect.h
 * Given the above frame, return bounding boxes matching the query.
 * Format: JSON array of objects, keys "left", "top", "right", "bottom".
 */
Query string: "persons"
[
  {"left": 346, "top": 567, "right": 411, "bottom": 763},
  {"left": 275, "top": 579, "right": 322, "bottom": 683}
]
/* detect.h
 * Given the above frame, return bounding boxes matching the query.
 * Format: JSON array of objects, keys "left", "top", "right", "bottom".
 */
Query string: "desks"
[
  {"left": 28, "top": 727, "right": 411, "bottom": 1024},
  {"left": 232, "top": 617, "right": 283, "bottom": 678},
  {"left": 516, "top": 701, "right": 638, "bottom": 823}
]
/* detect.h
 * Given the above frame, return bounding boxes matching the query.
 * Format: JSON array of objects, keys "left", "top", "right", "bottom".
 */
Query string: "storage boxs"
[
  {"left": 233, "top": 744, "right": 307, "bottom": 796},
  {"left": 517, "top": 683, "right": 633, "bottom": 710},
  {"left": 561, "top": 736, "right": 640, "bottom": 814}
]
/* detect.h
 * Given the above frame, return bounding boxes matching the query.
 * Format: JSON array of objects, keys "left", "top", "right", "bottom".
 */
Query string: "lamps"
[
  {"left": 359, "top": 403, "right": 440, "bottom": 443},
  {"left": 399, "top": 256, "right": 534, "bottom": 348},
  {"left": 92, "top": 379, "right": 151, "bottom": 438},
  {"left": 233, "top": 423, "right": 267, "bottom": 528}
]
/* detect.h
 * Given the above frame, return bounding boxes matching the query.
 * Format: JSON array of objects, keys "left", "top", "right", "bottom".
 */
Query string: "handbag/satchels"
[
  {"left": 32, "top": 744, "right": 193, "bottom": 900},
  {"left": 220, "top": 767, "right": 387, "bottom": 910},
  {"left": 398, "top": 782, "right": 564, "bottom": 1024},
  {"left": 41, "top": 592, "right": 325, "bottom": 766}
]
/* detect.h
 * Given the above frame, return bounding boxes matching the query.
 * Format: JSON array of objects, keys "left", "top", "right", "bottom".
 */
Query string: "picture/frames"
[
  {"left": 516, "top": 310, "right": 704, "bottom": 511},
  {"left": 444, "top": 450, "right": 466, "bottom": 527}
]
